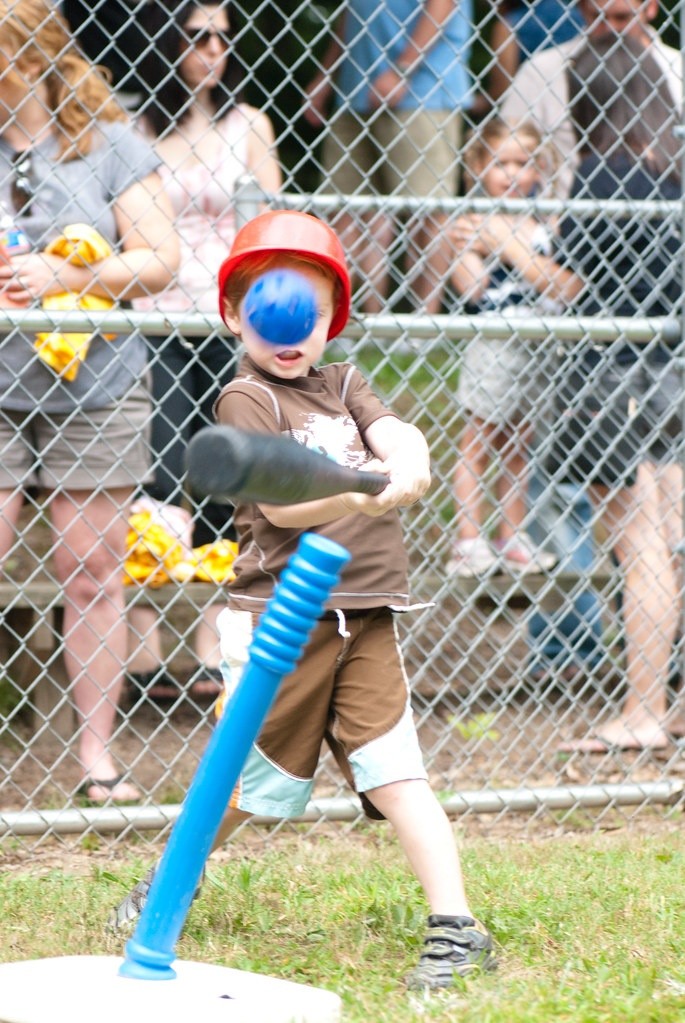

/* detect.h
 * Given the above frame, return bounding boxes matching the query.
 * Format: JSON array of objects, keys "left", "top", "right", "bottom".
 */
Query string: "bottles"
[{"left": 0, "top": 214, "right": 33, "bottom": 310}]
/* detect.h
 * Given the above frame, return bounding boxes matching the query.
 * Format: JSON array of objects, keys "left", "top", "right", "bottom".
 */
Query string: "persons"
[
  {"left": 102, "top": 205, "right": 503, "bottom": 989},
  {"left": 458, "top": 32, "right": 682, "bottom": 757},
  {"left": 433, "top": 119, "right": 563, "bottom": 579},
  {"left": 470, "top": 0, "right": 593, "bottom": 117},
  {"left": 464, "top": 0, "right": 685, "bottom": 229},
  {"left": 300, "top": 0, "right": 477, "bottom": 355},
  {"left": 112, "top": 2, "right": 283, "bottom": 706},
  {"left": 0, "top": 0, "right": 184, "bottom": 800}
]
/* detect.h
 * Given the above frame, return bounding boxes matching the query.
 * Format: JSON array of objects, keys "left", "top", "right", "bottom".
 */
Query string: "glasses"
[
  {"left": 581, "top": 7, "right": 650, "bottom": 20},
  {"left": 175, "top": 29, "right": 235, "bottom": 50}
]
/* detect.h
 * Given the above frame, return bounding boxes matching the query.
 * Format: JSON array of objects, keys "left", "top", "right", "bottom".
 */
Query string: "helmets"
[{"left": 219, "top": 210, "right": 352, "bottom": 343}]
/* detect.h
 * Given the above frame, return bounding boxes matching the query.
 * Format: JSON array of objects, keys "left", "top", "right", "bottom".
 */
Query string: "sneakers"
[
  {"left": 106, "top": 857, "right": 206, "bottom": 940},
  {"left": 408, "top": 912, "right": 502, "bottom": 995},
  {"left": 444, "top": 534, "right": 502, "bottom": 580},
  {"left": 489, "top": 533, "right": 559, "bottom": 572}
]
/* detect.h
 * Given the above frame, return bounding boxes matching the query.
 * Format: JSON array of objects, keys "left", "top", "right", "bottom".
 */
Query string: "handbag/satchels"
[{"left": 543, "top": 359, "right": 640, "bottom": 487}]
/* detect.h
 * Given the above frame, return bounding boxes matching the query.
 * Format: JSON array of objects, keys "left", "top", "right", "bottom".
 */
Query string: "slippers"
[
  {"left": 77, "top": 775, "right": 142, "bottom": 807},
  {"left": 556, "top": 728, "right": 666, "bottom": 756}
]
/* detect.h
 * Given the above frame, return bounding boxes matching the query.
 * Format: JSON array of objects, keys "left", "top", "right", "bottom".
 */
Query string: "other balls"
[{"left": 244, "top": 269, "right": 317, "bottom": 343}]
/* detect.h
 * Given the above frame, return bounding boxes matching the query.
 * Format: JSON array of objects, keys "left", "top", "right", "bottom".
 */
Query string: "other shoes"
[
  {"left": 192, "top": 665, "right": 224, "bottom": 698},
  {"left": 125, "top": 662, "right": 181, "bottom": 707}
]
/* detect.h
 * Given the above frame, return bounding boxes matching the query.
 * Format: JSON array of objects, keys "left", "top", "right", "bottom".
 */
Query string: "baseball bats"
[{"left": 184, "top": 423, "right": 389, "bottom": 502}]
